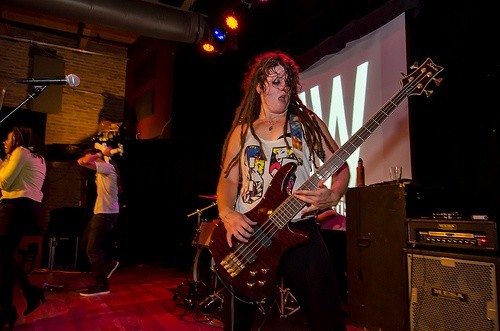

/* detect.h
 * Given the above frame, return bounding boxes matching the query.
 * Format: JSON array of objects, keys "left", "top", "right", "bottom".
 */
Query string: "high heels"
[
  {"left": 0, "top": 307, "right": 17, "bottom": 329},
  {"left": 23, "top": 286, "right": 46, "bottom": 315}
]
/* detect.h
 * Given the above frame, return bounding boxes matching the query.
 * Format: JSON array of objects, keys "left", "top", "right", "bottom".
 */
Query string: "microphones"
[{"left": 14, "top": 73, "right": 81, "bottom": 88}]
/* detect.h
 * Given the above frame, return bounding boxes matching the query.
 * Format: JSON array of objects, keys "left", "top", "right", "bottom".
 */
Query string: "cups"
[{"left": 389, "top": 166, "right": 402, "bottom": 182}]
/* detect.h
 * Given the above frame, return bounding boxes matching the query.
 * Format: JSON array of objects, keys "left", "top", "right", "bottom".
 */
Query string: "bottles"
[{"left": 356, "top": 158, "right": 364, "bottom": 186}]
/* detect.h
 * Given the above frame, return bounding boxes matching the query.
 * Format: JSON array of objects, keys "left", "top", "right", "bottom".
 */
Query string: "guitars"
[{"left": 206, "top": 54, "right": 444, "bottom": 307}]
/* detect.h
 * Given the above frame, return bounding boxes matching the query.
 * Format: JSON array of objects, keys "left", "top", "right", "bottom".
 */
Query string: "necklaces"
[{"left": 261, "top": 114, "right": 286, "bottom": 131}]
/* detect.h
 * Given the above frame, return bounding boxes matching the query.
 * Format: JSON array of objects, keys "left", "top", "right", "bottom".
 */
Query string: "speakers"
[
  {"left": 345, "top": 181, "right": 423, "bottom": 330},
  {"left": 401, "top": 247, "right": 500, "bottom": 330}
]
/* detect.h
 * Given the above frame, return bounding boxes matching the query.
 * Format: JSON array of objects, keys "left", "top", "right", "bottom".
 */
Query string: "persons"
[
  {"left": 78, "top": 147, "right": 119, "bottom": 296},
  {"left": 217, "top": 54, "right": 350, "bottom": 331},
  {"left": 0, "top": 126, "right": 47, "bottom": 331}
]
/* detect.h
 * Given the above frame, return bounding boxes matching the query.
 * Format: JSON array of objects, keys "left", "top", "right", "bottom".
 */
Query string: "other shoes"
[
  {"left": 80, "top": 285, "right": 109, "bottom": 296},
  {"left": 107, "top": 259, "right": 119, "bottom": 278}
]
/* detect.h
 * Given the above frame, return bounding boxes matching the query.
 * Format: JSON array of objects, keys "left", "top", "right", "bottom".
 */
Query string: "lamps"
[{"left": 202, "top": 0, "right": 251, "bottom": 52}]
